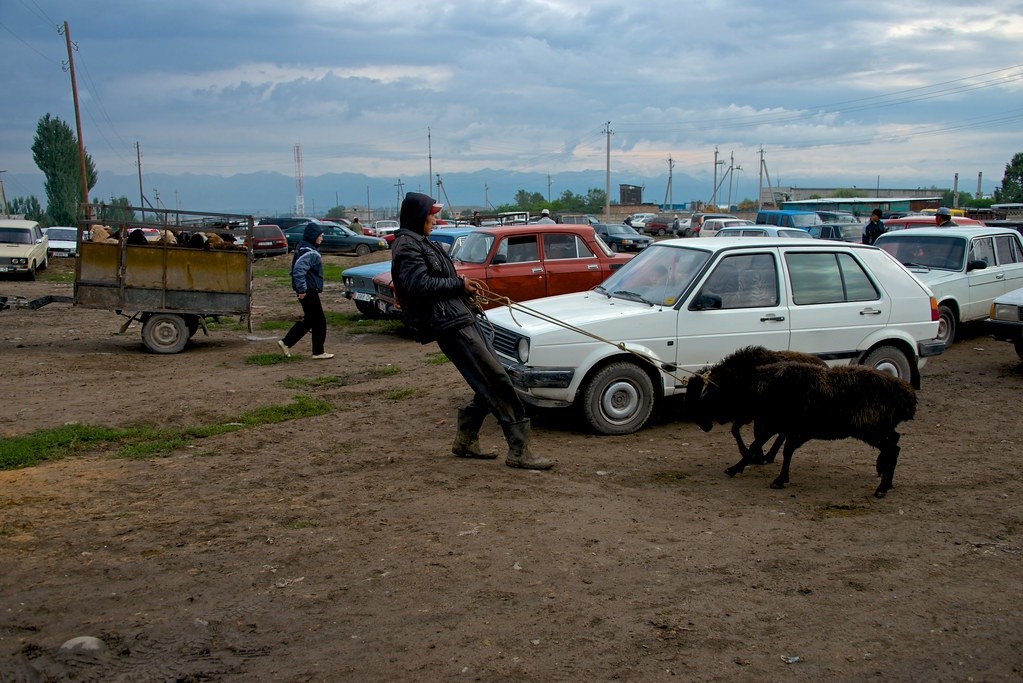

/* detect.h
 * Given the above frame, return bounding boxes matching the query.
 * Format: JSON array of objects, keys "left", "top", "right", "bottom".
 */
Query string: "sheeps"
[
  {"left": 717, "top": 358, "right": 917, "bottom": 498},
  {"left": 90, "top": 225, "right": 120, "bottom": 244},
  {"left": 155, "top": 230, "right": 178, "bottom": 247},
  {"left": 107, "top": 229, "right": 149, "bottom": 245},
  {"left": 188, "top": 232, "right": 248, "bottom": 250},
  {"left": 679, "top": 347, "right": 832, "bottom": 464}
]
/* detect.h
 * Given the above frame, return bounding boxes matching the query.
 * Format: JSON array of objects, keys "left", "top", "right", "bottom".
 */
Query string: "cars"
[
  {"left": 283, "top": 219, "right": 400, "bottom": 257},
  {"left": 343, "top": 211, "right": 1023, "bottom": 437},
  {"left": 243, "top": 225, "right": 289, "bottom": 256},
  {"left": 125, "top": 227, "right": 163, "bottom": 243},
  {"left": 0, "top": 220, "right": 89, "bottom": 280}
]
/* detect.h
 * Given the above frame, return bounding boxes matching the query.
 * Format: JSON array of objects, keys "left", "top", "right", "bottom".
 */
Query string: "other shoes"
[
  {"left": 312, "top": 352, "right": 334, "bottom": 359},
  {"left": 277, "top": 340, "right": 291, "bottom": 358}
]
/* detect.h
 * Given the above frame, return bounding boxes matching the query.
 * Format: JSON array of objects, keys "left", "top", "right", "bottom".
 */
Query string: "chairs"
[{"left": 723, "top": 268, "right": 772, "bottom": 308}]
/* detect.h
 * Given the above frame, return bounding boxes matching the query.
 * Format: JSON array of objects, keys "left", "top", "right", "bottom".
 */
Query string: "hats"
[
  {"left": 428, "top": 203, "right": 443, "bottom": 215},
  {"left": 542, "top": 209, "right": 549, "bottom": 214},
  {"left": 934, "top": 207, "right": 951, "bottom": 215},
  {"left": 674, "top": 215, "right": 678, "bottom": 218},
  {"left": 873, "top": 209, "right": 882, "bottom": 217}
]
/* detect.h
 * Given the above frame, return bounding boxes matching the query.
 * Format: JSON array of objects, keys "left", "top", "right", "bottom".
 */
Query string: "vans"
[{"left": 258, "top": 217, "right": 320, "bottom": 231}]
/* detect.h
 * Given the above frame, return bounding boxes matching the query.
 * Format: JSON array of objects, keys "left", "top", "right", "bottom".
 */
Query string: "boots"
[
  {"left": 452, "top": 406, "right": 499, "bottom": 459},
  {"left": 502, "top": 417, "right": 556, "bottom": 470}
]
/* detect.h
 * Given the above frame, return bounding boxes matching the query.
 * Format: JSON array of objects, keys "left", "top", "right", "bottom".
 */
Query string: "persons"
[
  {"left": 862, "top": 208, "right": 887, "bottom": 245},
  {"left": 935, "top": 207, "right": 959, "bottom": 227},
  {"left": 277, "top": 223, "right": 335, "bottom": 360},
  {"left": 470, "top": 211, "right": 481, "bottom": 227},
  {"left": 672, "top": 215, "right": 680, "bottom": 239},
  {"left": 625, "top": 215, "right": 632, "bottom": 226},
  {"left": 538, "top": 208, "right": 556, "bottom": 224},
  {"left": 390, "top": 192, "right": 555, "bottom": 471},
  {"left": 347, "top": 218, "right": 363, "bottom": 235}
]
